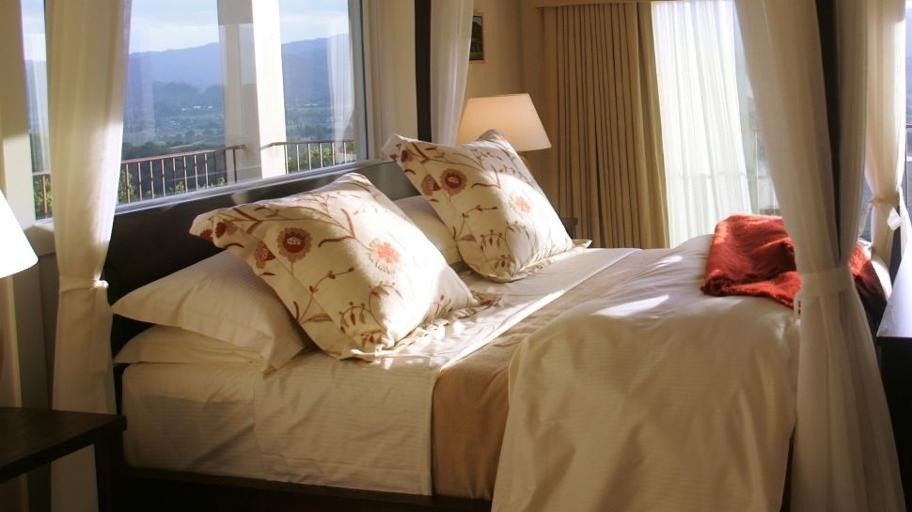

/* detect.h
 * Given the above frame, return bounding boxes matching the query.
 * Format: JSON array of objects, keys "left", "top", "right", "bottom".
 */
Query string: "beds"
[{"left": 100, "top": 161, "right": 891, "bottom": 510}]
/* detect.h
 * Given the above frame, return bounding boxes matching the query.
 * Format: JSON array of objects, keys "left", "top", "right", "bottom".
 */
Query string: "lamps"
[{"left": 454, "top": 93, "right": 553, "bottom": 152}]
